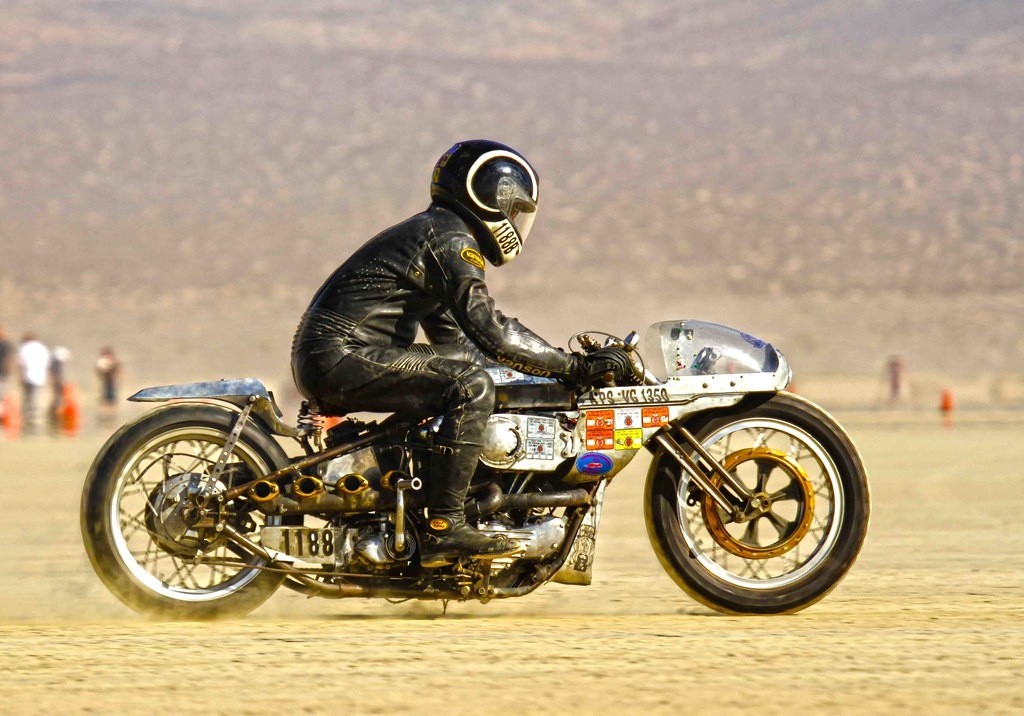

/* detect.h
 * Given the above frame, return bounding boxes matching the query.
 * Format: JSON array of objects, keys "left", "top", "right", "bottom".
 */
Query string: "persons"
[
  {"left": 0, "top": 327, "right": 121, "bottom": 439},
  {"left": 290, "top": 139, "right": 644, "bottom": 569}
]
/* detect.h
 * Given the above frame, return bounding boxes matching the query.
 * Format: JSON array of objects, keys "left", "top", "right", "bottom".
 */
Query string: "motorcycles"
[{"left": 77, "top": 320, "right": 870, "bottom": 618}]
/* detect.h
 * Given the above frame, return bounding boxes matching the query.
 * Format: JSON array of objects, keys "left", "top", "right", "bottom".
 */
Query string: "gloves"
[{"left": 564, "top": 349, "right": 636, "bottom": 390}]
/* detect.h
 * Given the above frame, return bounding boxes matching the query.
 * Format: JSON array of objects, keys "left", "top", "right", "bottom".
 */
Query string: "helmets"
[{"left": 430, "top": 139, "right": 540, "bottom": 269}]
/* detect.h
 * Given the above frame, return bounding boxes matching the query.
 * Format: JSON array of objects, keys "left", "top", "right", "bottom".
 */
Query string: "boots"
[{"left": 418, "top": 433, "right": 528, "bottom": 567}]
